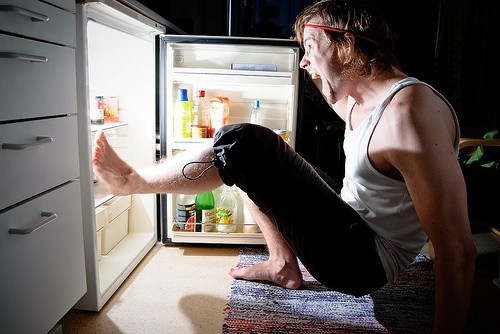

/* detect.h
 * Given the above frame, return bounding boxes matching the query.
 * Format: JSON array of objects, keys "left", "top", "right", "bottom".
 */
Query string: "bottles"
[
  {"left": 250, "top": 99, "right": 262, "bottom": 125},
  {"left": 195, "top": 190, "right": 216, "bottom": 232},
  {"left": 173, "top": 88, "right": 192, "bottom": 139},
  {"left": 191, "top": 90, "right": 211, "bottom": 138},
  {"left": 215, "top": 184, "right": 238, "bottom": 233},
  {"left": 175, "top": 193, "right": 196, "bottom": 231}
]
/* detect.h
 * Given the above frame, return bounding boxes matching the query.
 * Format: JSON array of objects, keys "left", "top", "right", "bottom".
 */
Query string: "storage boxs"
[
  {"left": 95, "top": 208, "right": 105, "bottom": 261},
  {"left": 101, "top": 194, "right": 132, "bottom": 255}
]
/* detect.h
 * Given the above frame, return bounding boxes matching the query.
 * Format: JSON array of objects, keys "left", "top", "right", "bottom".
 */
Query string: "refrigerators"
[{"left": 72, "top": 0, "right": 300, "bottom": 312}]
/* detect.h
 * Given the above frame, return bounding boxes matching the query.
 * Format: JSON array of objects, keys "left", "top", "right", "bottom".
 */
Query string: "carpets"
[{"left": 223, "top": 244, "right": 436, "bottom": 334}]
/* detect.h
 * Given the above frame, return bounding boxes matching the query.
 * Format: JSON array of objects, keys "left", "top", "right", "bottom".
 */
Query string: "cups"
[
  {"left": 208, "top": 96, "right": 231, "bottom": 137},
  {"left": 90, "top": 94, "right": 119, "bottom": 126}
]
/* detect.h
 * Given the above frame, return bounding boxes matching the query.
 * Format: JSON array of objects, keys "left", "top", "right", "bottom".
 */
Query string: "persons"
[{"left": 92, "top": 1, "right": 476, "bottom": 334}]
